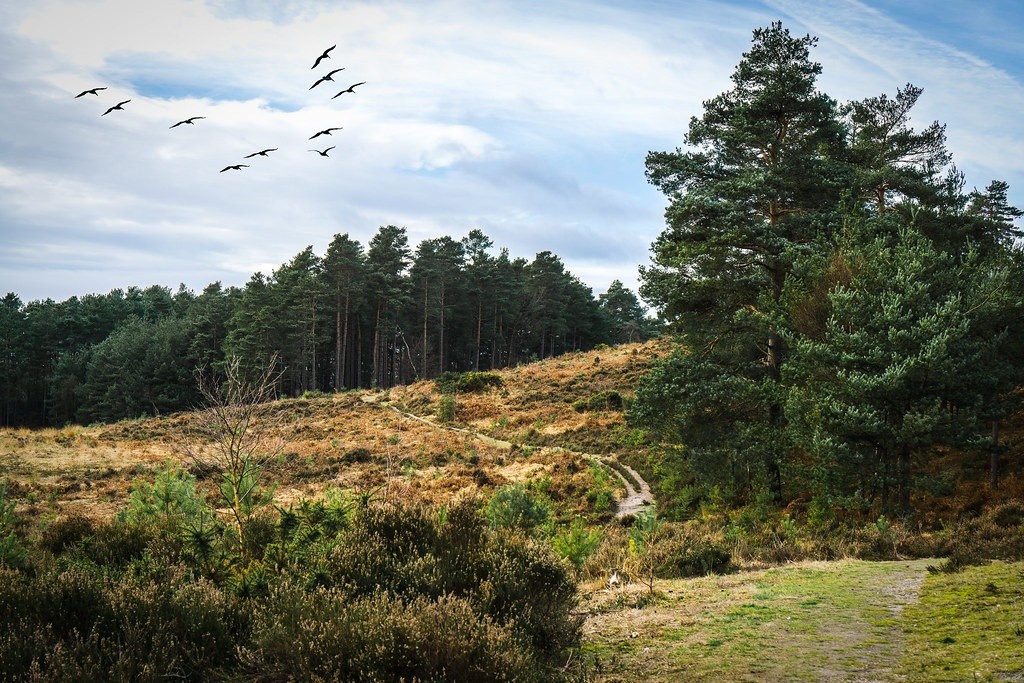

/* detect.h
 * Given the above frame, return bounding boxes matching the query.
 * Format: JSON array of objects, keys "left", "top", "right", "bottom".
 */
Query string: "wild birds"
[
  {"left": 219, "top": 148, "right": 279, "bottom": 173},
  {"left": 74, "top": 87, "right": 131, "bottom": 117},
  {"left": 307, "top": 127, "right": 343, "bottom": 158},
  {"left": 308, "top": 44, "right": 366, "bottom": 100},
  {"left": 169, "top": 117, "right": 206, "bottom": 130}
]
